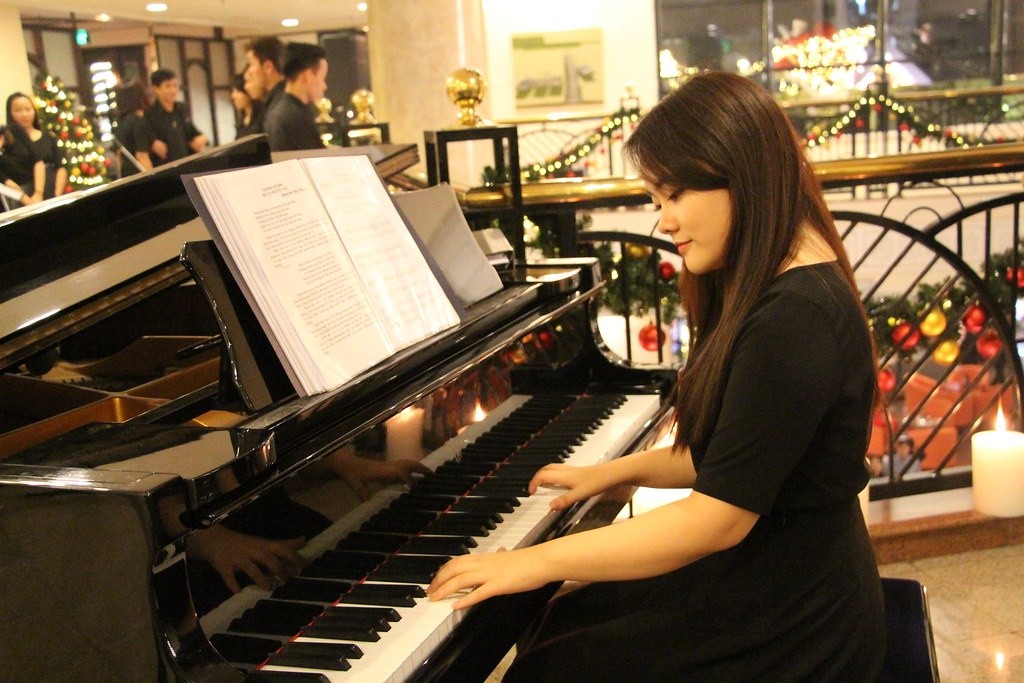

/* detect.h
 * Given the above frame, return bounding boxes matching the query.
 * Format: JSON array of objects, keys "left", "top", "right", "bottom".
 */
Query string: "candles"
[
  {"left": 971, "top": 397, "right": 1024, "bottom": 518},
  {"left": 384, "top": 406, "right": 424, "bottom": 461}
]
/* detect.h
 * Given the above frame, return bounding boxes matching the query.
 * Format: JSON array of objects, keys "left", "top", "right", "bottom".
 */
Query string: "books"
[{"left": 172, "top": 153, "right": 461, "bottom": 398}]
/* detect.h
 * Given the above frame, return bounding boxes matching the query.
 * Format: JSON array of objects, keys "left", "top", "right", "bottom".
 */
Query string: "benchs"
[{"left": 881, "top": 578, "right": 940, "bottom": 683}]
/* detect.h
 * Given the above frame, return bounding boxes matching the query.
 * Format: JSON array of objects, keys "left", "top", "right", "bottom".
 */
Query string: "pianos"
[{"left": 0, "top": 132, "right": 686, "bottom": 683}]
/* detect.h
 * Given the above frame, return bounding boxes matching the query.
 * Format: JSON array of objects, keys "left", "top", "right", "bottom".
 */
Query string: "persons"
[
  {"left": 1, "top": 35, "right": 331, "bottom": 215},
  {"left": 156, "top": 433, "right": 436, "bottom": 595},
  {"left": 427, "top": 69, "right": 882, "bottom": 683}
]
